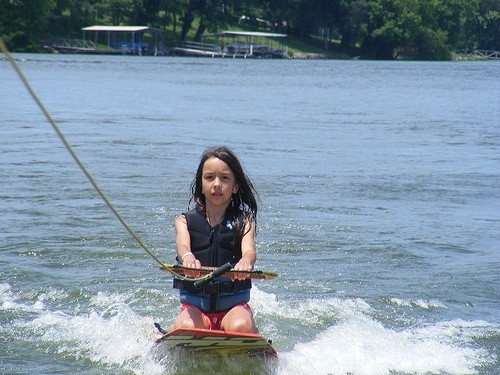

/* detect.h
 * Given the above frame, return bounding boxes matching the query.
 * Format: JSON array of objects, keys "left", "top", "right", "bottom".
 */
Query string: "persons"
[{"left": 167, "top": 146, "right": 260, "bottom": 333}]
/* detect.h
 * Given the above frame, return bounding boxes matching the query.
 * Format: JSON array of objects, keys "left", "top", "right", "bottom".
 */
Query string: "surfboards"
[{"left": 155, "top": 327, "right": 278, "bottom": 359}]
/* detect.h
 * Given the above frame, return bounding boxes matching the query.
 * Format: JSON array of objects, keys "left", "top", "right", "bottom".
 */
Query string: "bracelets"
[{"left": 178, "top": 252, "right": 193, "bottom": 260}]
